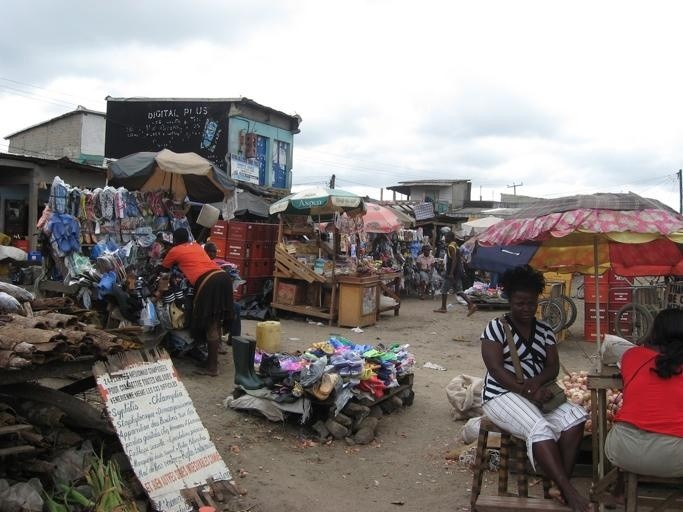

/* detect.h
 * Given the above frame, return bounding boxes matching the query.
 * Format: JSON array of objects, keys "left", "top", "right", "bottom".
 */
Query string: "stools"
[{"left": 469, "top": 418, "right": 552, "bottom": 508}]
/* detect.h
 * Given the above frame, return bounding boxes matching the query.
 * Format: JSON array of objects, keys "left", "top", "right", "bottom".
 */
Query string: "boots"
[
  {"left": 194, "top": 339, "right": 219, "bottom": 376},
  {"left": 229, "top": 335, "right": 259, "bottom": 391},
  {"left": 241, "top": 335, "right": 265, "bottom": 387}
]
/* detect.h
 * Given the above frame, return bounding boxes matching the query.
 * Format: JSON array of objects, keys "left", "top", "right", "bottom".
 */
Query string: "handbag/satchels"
[
  {"left": 600, "top": 333, "right": 639, "bottom": 367},
  {"left": 529, "top": 378, "right": 567, "bottom": 415}
]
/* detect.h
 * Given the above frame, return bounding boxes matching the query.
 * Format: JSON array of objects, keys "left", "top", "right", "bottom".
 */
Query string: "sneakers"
[
  {"left": 433, "top": 307, "right": 447, "bottom": 313},
  {"left": 259, "top": 351, "right": 290, "bottom": 378},
  {"left": 466, "top": 303, "right": 479, "bottom": 316}
]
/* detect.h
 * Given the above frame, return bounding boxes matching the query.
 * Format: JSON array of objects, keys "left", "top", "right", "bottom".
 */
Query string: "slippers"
[
  {"left": 48, "top": 182, "right": 196, "bottom": 330},
  {"left": 278, "top": 336, "right": 414, "bottom": 400},
  {"left": 393, "top": 229, "right": 424, "bottom": 275}
]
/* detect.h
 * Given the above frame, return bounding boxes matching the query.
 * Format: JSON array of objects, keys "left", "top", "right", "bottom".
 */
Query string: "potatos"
[{"left": 557, "top": 371, "right": 624, "bottom": 432}]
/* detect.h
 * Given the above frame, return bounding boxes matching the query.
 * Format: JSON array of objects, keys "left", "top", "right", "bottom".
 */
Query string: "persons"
[
  {"left": 416, "top": 231, "right": 476, "bottom": 317},
  {"left": 604, "top": 308, "right": 683, "bottom": 478},
  {"left": 161, "top": 228, "right": 241, "bottom": 376},
  {"left": 481, "top": 264, "right": 590, "bottom": 512}
]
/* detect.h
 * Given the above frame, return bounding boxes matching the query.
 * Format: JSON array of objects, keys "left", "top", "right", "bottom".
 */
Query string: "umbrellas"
[
  {"left": 107, "top": 149, "right": 238, "bottom": 204},
  {"left": 459, "top": 194, "right": 683, "bottom": 491},
  {"left": 269, "top": 186, "right": 416, "bottom": 259}
]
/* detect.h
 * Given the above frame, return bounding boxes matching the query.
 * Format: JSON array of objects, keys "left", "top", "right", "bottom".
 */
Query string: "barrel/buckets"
[{"left": 255, "top": 320, "right": 281, "bottom": 354}]
